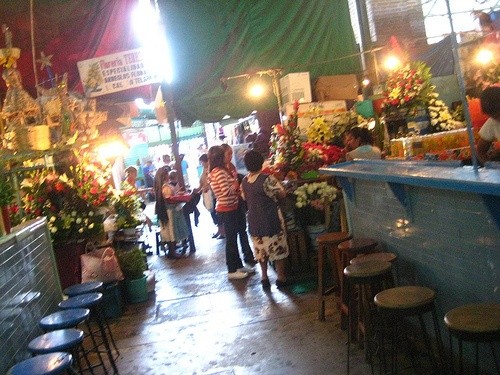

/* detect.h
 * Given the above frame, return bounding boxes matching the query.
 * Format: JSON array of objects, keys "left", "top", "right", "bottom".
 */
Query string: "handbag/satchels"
[{"left": 81, "top": 241, "right": 125, "bottom": 287}]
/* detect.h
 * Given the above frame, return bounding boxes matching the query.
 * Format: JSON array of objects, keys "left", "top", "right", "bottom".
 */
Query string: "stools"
[
  {"left": 156, "top": 232, "right": 166, "bottom": 255},
  {"left": 8, "top": 280, "right": 121, "bottom": 375},
  {"left": 308, "top": 232, "right": 500, "bottom": 375}
]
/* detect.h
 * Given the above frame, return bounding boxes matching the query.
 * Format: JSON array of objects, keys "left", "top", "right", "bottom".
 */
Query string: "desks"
[{"left": 318, "top": 158, "right": 500, "bottom": 375}]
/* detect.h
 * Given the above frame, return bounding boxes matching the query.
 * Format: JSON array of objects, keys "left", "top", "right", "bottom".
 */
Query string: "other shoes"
[
  {"left": 228, "top": 266, "right": 256, "bottom": 280},
  {"left": 260, "top": 277, "right": 269, "bottom": 285},
  {"left": 212, "top": 233, "right": 225, "bottom": 240},
  {"left": 277, "top": 278, "right": 296, "bottom": 286}
]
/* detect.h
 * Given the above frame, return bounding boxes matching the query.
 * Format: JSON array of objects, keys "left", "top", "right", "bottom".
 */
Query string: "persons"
[
  {"left": 143, "top": 153, "right": 190, "bottom": 201},
  {"left": 122, "top": 165, "right": 150, "bottom": 210},
  {"left": 154, "top": 164, "right": 183, "bottom": 258},
  {"left": 476, "top": 86, "right": 500, "bottom": 170},
  {"left": 199, "top": 153, "right": 225, "bottom": 239},
  {"left": 220, "top": 143, "right": 258, "bottom": 263},
  {"left": 207, "top": 145, "right": 255, "bottom": 279},
  {"left": 344, "top": 127, "right": 382, "bottom": 161},
  {"left": 241, "top": 150, "right": 299, "bottom": 289}
]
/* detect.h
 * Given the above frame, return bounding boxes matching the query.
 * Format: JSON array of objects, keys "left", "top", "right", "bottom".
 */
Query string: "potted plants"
[{"left": 117, "top": 245, "right": 148, "bottom": 303}]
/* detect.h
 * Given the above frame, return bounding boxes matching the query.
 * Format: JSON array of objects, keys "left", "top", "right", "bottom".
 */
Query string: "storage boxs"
[
  {"left": 278, "top": 71, "right": 312, "bottom": 106},
  {"left": 312, "top": 74, "right": 358, "bottom": 101},
  {"left": 282, "top": 100, "right": 346, "bottom": 130}
]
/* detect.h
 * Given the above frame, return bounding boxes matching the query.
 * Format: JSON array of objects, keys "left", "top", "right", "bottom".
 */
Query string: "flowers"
[
  {"left": 294, "top": 180, "right": 337, "bottom": 225},
  {"left": 10, "top": 165, "right": 140, "bottom": 239},
  {"left": 264, "top": 54, "right": 464, "bottom": 173}
]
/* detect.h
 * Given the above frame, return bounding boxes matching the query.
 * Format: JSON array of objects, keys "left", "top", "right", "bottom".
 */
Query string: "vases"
[
  {"left": 123, "top": 226, "right": 136, "bottom": 242},
  {"left": 309, "top": 224, "right": 326, "bottom": 250}
]
